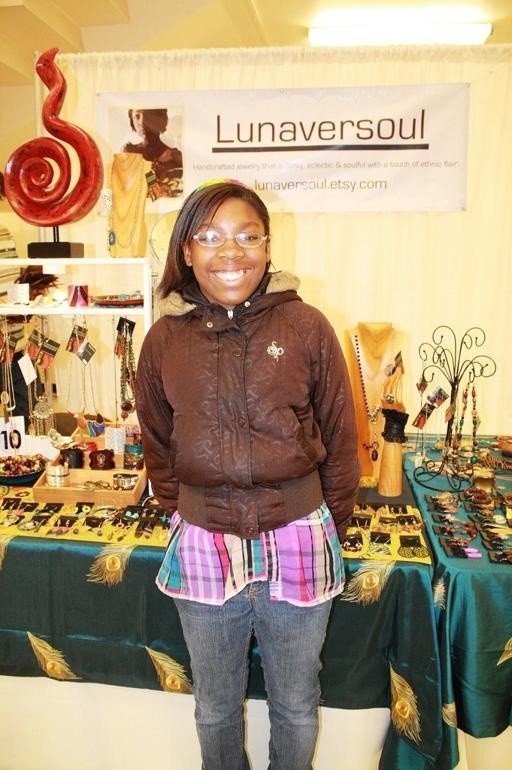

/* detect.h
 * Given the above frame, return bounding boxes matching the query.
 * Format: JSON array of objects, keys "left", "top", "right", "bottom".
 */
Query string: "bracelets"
[{"left": 444, "top": 516, "right": 477, "bottom": 543}]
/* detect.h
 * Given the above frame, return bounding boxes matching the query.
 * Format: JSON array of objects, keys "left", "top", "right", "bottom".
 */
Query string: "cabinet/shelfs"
[{"left": 1, "top": 253, "right": 166, "bottom": 343}]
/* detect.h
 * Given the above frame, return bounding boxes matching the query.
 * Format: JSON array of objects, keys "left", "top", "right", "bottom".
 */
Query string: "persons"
[
  {"left": 132, "top": 186, "right": 360, "bottom": 769},
  {"left": 107, "top": 110, "right": 182, "bottom": 258}
]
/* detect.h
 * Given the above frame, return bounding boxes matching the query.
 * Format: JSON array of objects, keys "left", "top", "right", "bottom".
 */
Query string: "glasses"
[{"left": 192, "top": 230, "right": 268, "bottom": 250}]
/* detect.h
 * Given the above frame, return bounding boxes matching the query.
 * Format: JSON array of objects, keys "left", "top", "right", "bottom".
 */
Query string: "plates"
[
  {"left": 90, "top": 294, "right": 144, "bottom": 306},
  {"left": 0, "top": 469, "right": 45, "bottom": 487}
]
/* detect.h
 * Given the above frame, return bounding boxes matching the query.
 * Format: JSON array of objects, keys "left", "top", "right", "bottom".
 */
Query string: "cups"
[
  {"left": 44, "top": 463, "right": 71, "bottom": 489},
  {"left": 124, "top": 443, "right": 144, "bottom": 470},
  {"left": 67, "top": 283, "right": 88, "bottom": 307}
]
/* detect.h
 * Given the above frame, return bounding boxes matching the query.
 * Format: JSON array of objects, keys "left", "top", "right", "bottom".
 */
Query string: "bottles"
[{"left": 414, "top": 452, "right": 422, "bottom": 468}]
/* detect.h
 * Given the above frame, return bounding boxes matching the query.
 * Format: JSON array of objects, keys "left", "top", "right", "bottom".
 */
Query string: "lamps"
[{"left": 304, "top": 4, "right": 494, "bottom": 49}]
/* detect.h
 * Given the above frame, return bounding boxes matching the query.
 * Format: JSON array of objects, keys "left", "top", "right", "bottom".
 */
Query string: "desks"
[
  {"left": 396, "top": 432, "right": 511, "bottom": 770},
  {"left": 0, "top": 408, "right": 451, "bottom": 770}
]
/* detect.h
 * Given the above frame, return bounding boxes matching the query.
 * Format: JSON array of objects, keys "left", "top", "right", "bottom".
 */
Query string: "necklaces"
[
  {"left": 414, "top": 381, "right": 480, "bottom": 477},
  {"left": 0, "top": 316, "right": 137, "bottom": 440},
  {"left": 353, "top": 332, "right": 400, "bottom": 461}
]
